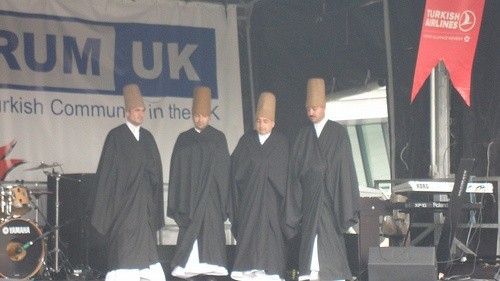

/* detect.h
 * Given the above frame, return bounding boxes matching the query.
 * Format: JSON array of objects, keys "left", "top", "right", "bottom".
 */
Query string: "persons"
[
  {"left": 226, "top": 92, "right": 303, "bottom": 281},
  {"left": 167, "top": 86, "right": 231, "bottom": 279},
  {"left": 283, "top": 78, "right": 362, "bottom": 281},
  {"left": 89, "top": 84, "right": 166, "bottom": 281}
]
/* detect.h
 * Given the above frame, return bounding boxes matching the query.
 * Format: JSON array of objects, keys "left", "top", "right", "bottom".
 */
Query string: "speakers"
[
  {"left": 369, "top": 247, "right": 438, "bottom": 281},
  {"left": 47, "top": 173, "right": 111, "bottom": 281}
]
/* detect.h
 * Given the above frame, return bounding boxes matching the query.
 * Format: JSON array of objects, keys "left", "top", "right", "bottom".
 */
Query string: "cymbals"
[
  {"left": 25, "top": 164, "right": 63, "bottom": 171},
  {"left": 29, "top": 190, "right": 54, "bottom": 198}
]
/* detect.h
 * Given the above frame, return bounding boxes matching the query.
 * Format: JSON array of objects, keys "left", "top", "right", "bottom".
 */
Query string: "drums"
[
  {"left": 2, "top": 185, "right": 32, "bottom": 215},
  {"left": 1, "top": 218, "right": 45, "bottom": 280}
]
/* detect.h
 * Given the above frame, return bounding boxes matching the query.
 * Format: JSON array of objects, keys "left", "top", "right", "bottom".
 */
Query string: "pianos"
[{"left": 387, "top": 155, "right": 495, "bottom": 279}]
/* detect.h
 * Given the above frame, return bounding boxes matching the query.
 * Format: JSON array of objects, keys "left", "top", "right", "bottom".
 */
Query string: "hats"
[
  {"left": 123, "top": 84, "right": 145, "bottom": 111},
  {"left": 305, "top": 79, "right": 325, "bottom": 108},
  {"left": 191, "top": 87, "right": 211, "bottom": 116},
  {"left": 255, "top": 92, "right": 276, "bottom": 122}
]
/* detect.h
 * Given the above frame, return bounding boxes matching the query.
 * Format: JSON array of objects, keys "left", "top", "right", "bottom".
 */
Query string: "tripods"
[{"left": 45, "top": 172, "right": 78, "bottom": 281}]
